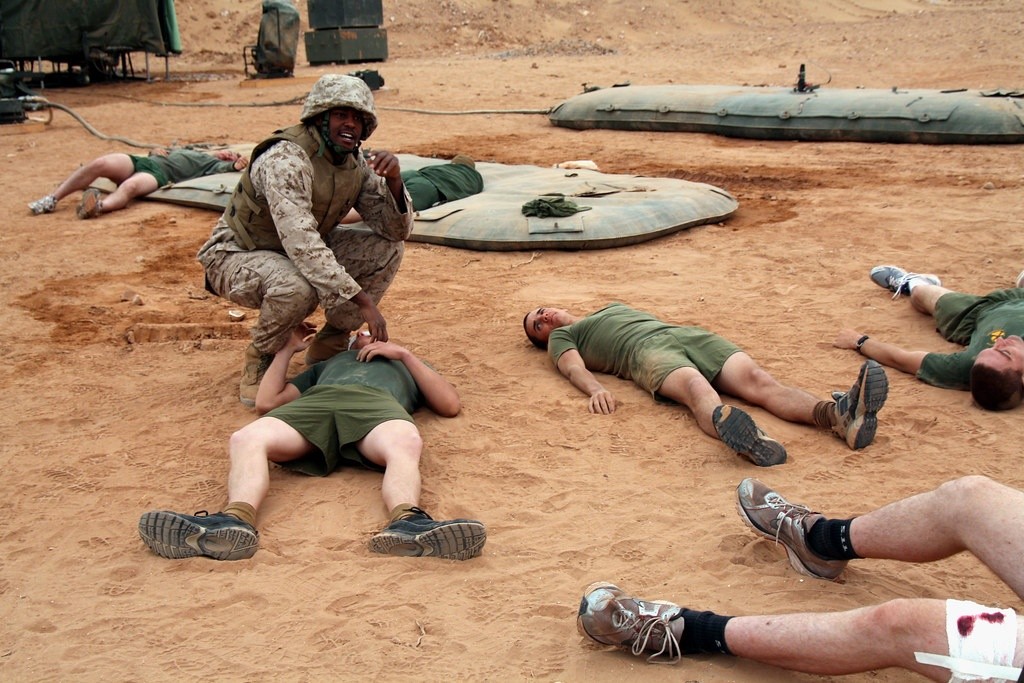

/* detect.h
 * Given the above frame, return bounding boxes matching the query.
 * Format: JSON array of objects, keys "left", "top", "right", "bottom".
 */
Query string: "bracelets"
[
  {"left": 854, "top": 335, "right": 869, "bottom": 356},
  {"left": 232, "top": 161, "right": 240, "bottom": 172}
]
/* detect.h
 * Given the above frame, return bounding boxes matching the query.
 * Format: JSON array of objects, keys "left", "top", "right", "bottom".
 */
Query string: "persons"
[
  {"left": 195, "top": 69, "right": 413, "bottom": 412},
  {"left": 139, "top": 310, "right": 490, "bottom": 561},
  {"left": 333, "top": 152, "right": 490, "bottom": 226},
  {"left": 523, "top": 295, "right": 889, "bottom": 467},
  {"left": 833, "top": 265, "right": 1024, "bottom": 413},
  {"left": 578, "top": 475, "right": 1024, "bottom": 683},
  {"left": 29, "top": 139, "right": 247, "bottom": 222}
]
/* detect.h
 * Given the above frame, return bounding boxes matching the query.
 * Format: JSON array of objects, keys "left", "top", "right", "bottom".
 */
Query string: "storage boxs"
[{"left": 305, "top": 0, "right": 388, "bottom": 66}]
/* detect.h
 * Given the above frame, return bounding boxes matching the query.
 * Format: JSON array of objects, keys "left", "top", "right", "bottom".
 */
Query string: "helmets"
[{"left": 300, "top": 74, "right": 377, "bottom": 142}]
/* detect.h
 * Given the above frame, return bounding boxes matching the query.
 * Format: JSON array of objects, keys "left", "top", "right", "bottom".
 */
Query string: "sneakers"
[
  {"left": 239, "top": 345, "right": 270, "bottom": 404},
  {"left": 712, "top": 404, "right": 787, "bottom": 468},
  {"left": 305, "top": 323, "right": 350, "bottom": 364},
  {"left": 736, "top": 477, "right": 850, "bottom": 583},
  {"left": 830, "top": 359, "right": 889, "bottom": 451},
  {"left": 28, "top": 195, "right": 57, "bottom": 215},
  {"left": 75, "top": 188, "right": 100, "bottom": 220},
  {"left": 868, "top": 265, "right": 940, "bottom": 301},
  {"left": 137, "top": 508, "right": 258, "bottom": 560},
  {"left": 368, "top": 506, "right": 486, "bottom": 564},
  {"left": 578, "top": 582, "right": 691, "bottom": 666}
]
